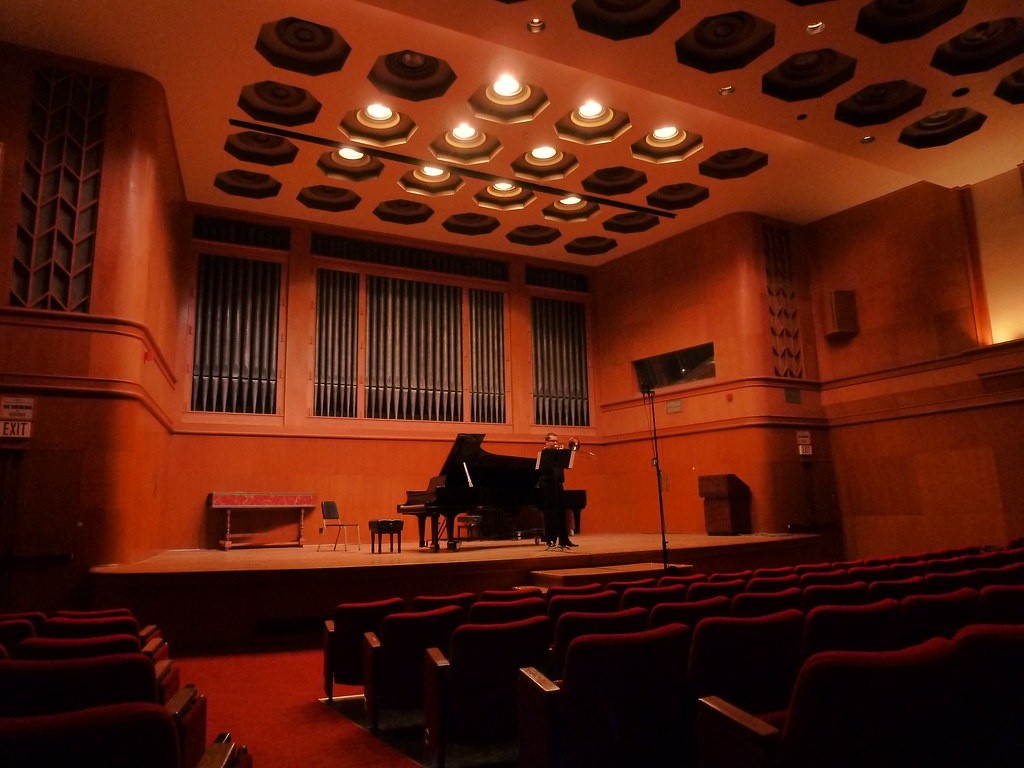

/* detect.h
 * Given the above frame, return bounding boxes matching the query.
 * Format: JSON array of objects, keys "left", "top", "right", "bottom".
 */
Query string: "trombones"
[{"left": 553, "top": 436, "right": 596, "bottom": 458}]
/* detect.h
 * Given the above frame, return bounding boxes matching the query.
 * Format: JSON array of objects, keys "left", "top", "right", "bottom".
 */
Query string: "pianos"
[{"left": 397, "top": 433, "right": 537, "bottom": 553}]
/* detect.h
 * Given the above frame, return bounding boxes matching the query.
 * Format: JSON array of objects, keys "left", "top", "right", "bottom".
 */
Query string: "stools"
[
  {"left": 368, "top": 519, "right": 404, "bottom": 553},
  {"left": 457, "top": 516, "right": 483, "bottom": 542}
]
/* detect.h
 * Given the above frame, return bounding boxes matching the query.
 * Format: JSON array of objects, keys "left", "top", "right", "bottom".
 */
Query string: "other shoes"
[
  {"left": 559, "top": 543, "right": 579, "bottom": 547},
  {"left": 547, "top": 546, "right": 563, "bottom": 551}
]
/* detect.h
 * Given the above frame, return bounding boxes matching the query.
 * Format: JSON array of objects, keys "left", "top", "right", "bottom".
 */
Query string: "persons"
[{"left": 539, "top": 433, "right": 579, "bottom": 547}]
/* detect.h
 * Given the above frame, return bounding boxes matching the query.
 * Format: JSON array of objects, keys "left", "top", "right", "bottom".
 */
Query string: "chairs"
[
  {"left": 325, "top": 537, "right": 1024, "bottom": 767},
  {"left": 317, "top": 499, "right": 361, "bottom": 551},
  {"left": 0, "top": 605, "right": 255, "bottom": 767}
]
